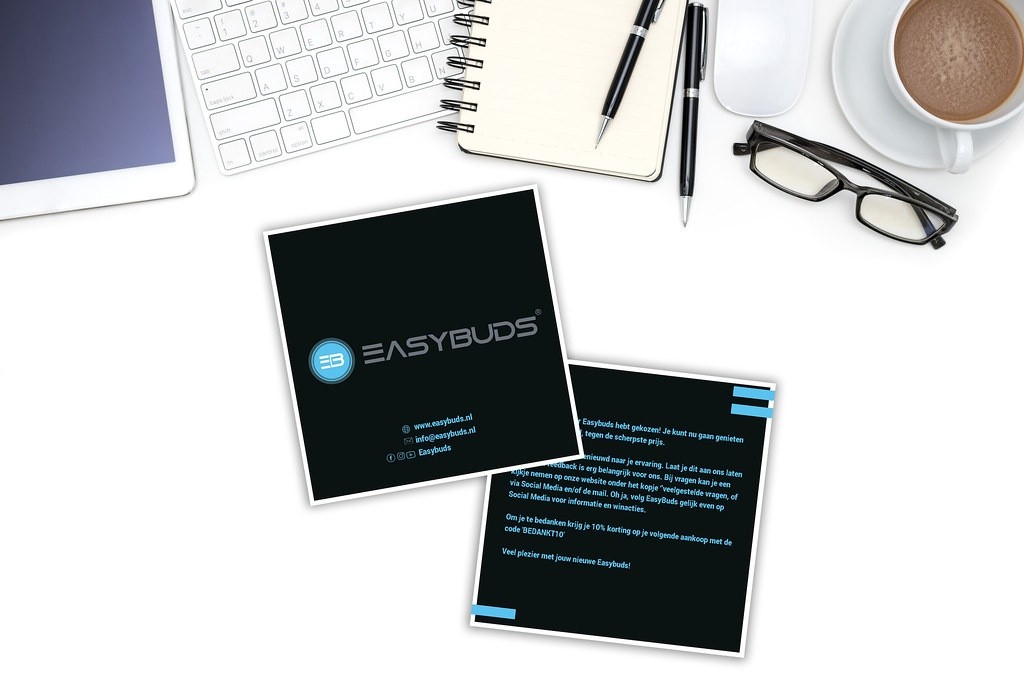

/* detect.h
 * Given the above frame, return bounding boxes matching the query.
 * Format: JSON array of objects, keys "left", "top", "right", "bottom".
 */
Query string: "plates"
[{"left": 830, "top": 0, "right": 1024, "bottom": 170}]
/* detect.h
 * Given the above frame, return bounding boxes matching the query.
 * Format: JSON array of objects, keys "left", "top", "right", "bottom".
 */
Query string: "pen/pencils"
[
  {"left": 591, "top": 0, "right": 664, "bottom": 153},
  {"left": 679, "top": 2, "right": 710, "bottom": 228}
]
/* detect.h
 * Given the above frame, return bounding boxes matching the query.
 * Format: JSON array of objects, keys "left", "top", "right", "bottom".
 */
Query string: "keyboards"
[{"left": 170, "top": 0, "right": 476, "bottom": 176}]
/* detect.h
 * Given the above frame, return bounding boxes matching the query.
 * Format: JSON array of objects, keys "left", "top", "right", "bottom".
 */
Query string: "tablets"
[{"left": 2, "top": 0, "right": 197, "bottom": 222}]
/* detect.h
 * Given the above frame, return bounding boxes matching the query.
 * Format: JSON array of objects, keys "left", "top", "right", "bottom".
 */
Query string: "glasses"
[{"left": 733, "top": 119, "right": 959, "bottom": 250}]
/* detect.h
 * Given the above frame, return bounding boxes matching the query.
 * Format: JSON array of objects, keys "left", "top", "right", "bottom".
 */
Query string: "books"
[{"left": 457, "top": 0, "right": 691, "bottom": 183}]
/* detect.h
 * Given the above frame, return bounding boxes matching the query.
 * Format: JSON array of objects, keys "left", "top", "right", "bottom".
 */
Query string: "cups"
[{"left": 883, "top": 0, "right": 1024, "bottom": 175}]
